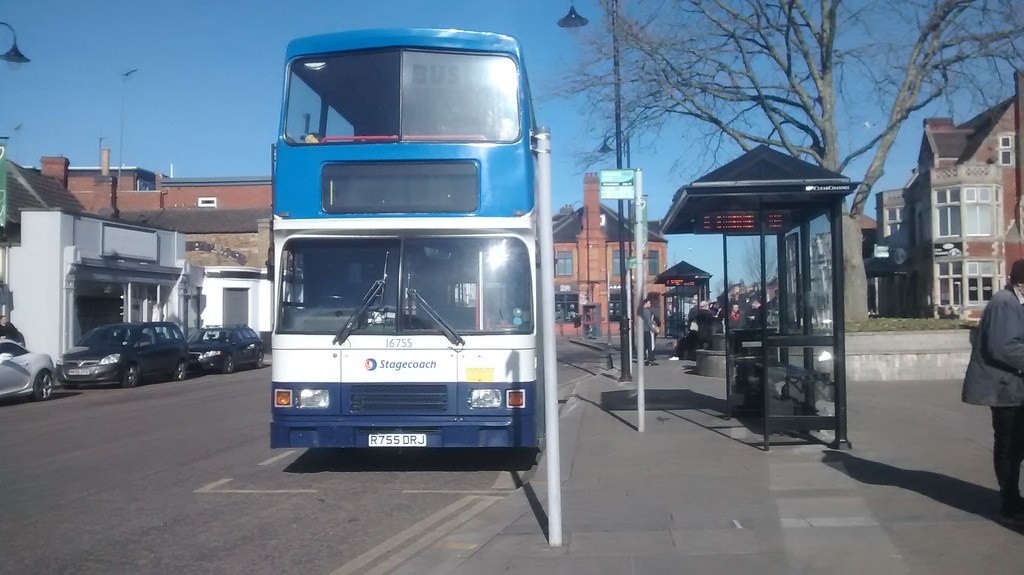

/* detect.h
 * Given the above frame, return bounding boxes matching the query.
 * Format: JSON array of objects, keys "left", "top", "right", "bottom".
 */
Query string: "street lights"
[
  {"left": 117, "top": 68, "right": 138, "bottom": 189},
  {"left": 599, "top": 133, "right": 637, "bottom": 358},
  {"left": 557, "top": 0, "right": 633, "bottom": 382}
]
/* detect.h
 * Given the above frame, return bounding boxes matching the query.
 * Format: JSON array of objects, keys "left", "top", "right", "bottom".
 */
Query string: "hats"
[{"left": 1010, "top": 259, "right": 1024, "bottom": 282}]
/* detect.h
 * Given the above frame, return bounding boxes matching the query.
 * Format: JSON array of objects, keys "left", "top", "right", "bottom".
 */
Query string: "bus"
[{"left": 266, "top": 25, "right": 541, "bottom": 449}]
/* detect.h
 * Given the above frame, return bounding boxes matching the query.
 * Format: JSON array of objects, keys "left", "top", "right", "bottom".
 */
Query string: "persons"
[
  {"left": 0, "top": 315, "right": 17, "bottom": 341},
  {"left": 643, "top": 299, "right": 661, "bottom": 366},
  {"left": 959, "top": 259, "right": 1024, "bottom": 525},
  {"left": 668, "top": 286, "right": 778, "bottom": 361}
]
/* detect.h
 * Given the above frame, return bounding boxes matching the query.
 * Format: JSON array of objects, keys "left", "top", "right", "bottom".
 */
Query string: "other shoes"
[
  {"left": 1002, "top": 495, "right": 1024, "bottom": 518},
  {"left": 645, "top": 360, "right": 659, "bottom": 366},
  {"left": 669, "top": 356, "right": 679, "bottom": 361}
]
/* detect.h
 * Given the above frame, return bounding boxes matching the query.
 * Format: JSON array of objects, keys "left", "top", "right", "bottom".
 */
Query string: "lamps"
[{"left": 184, "top": 292, "right": 190, "bottom": 301}]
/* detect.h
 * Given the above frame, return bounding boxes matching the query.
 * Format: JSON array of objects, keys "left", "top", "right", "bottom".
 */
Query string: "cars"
[
  {"left": 186, "top": 325, "right": 265, "bottom": 374},
  {"left": 0, "top": 337, "right": 56, "bottom": 402}
]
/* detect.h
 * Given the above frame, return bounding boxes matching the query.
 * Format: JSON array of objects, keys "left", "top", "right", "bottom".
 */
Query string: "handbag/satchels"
[{"left": 650, "top": 313, "right": 659, "bottom": 334}]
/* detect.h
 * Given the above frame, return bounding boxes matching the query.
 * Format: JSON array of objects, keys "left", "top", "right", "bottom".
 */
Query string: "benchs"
[{"left": 750, "top": 358, "right": 830, "bottom": 416}]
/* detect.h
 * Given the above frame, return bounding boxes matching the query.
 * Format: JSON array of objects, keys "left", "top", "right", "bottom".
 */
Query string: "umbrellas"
[{"left": 865, "top": 259, "right": 907, "bottom": 316}]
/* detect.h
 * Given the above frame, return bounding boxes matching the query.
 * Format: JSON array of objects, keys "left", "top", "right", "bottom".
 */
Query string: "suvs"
[{"left": 55, "top": 321, "right": 188, "bottom": 389}]
